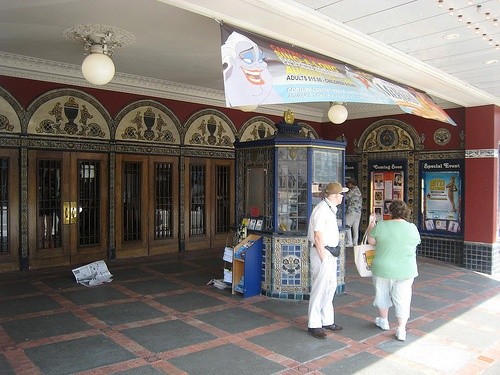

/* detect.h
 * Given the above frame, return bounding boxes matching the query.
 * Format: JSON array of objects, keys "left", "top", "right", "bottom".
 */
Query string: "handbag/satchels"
[
  {"left": 353, "top": 244, "right": 376, "bottom": 277},
  {"left": 325, "top": 246, "right": 340, "bottom": 257}
]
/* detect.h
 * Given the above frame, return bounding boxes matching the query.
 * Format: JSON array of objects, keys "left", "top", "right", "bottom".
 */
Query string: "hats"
[{"left": 325, "top": 183, "right": 349, "bottom": 193}]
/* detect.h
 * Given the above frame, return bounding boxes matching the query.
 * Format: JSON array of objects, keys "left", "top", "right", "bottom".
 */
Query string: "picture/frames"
[{"left": 248, "top": 217, "right": 265, "bottom": 231}]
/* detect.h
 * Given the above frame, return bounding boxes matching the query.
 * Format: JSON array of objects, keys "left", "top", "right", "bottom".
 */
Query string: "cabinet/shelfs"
[
  {"left": 232, "top": 233, "right": 263, "bottom": 298},
  {"left": 224, "top": 229, "right": 237, "bottom": 288}
]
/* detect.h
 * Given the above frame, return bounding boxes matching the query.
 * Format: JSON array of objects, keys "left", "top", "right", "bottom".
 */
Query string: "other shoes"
[
  {"left": 375, "top": 317, "right": 390, "bottom": 330},
  {"left": 346, "top": 244, "right": 353, "bottom": 247},
  {"left": 307, "top": 327, "right": 327, "bottom": 339},
  {"left": 395, "top": 328, "right": 406, "bottom": 341},
  {"left": 322, "top": 323, "right": 342, "bottom": 330}
]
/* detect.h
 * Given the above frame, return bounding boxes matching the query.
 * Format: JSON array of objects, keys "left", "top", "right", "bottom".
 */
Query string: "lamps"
[
  {"left": 75, "top": 30, "right": 122, "bottom": 85},
  {"left": 327, "top": 102, "right": 348, "bottom": 125}
]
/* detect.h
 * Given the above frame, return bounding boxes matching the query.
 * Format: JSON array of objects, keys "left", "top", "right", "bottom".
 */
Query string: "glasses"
[{"left": 339, "top": 192, "right": 343, "bottom": 195}]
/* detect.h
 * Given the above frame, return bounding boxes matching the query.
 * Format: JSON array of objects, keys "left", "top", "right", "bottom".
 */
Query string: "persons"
[
  {"left": 368, "top": 201, "right": 421, "bottom": 341},
  {"left": 307, "top": 182, "right": 350, "bottom": 339},
  {"left": 344, "top": 179, "right": 363, "bottom": 248}
]
